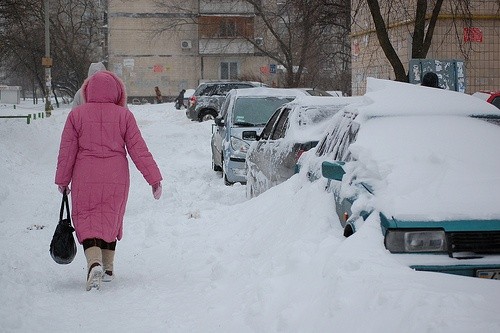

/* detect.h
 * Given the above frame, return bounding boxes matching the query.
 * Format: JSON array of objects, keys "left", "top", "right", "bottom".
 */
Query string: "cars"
[
  {"left": 294, "top": 103, "right": 500, "bottom": 280},
  {"left": 471, "top": 89, "right": 500, "bottom": 111},
  {"left": 182, "top": 88, "right": 196, "bottom": 109},
  {"left": 241, "top": 95, "right": 364, "bottom": 199},
  {"left": 211, "top": 87, "right": 334, "bottom": 187}
]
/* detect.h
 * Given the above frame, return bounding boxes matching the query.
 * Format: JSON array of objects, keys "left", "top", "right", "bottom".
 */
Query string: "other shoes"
[
  {"left": 85, "top": 263, "right": 103, "bottom": 292},
  {"left": 102, "top": 271, "right": 113, "bottom": 282}
]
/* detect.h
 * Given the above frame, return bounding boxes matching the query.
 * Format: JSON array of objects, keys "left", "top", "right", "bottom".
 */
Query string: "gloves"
[
  {"left": 152, "top": 182, "right": 162, "bottom": 200},
  {"left": 58, "top": 185, "right": 71, "bottom": 195}
]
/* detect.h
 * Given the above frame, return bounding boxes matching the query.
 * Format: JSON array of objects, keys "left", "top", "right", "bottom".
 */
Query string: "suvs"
[{"left": 185, "top": 80, "right": 271, "bottom": 123}]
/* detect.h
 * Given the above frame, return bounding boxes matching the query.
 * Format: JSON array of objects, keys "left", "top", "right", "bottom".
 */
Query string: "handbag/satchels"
[{"left": 49, "top": 189, "right": 77, "bottom": 264}]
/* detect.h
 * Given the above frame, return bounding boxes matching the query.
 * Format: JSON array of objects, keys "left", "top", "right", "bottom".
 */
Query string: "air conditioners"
[
  {"left": 181, "top": 39, "right": 192, "bottom": 51},
  {"left": 255, "top": 37, "right": 264, "bottom": 46}
]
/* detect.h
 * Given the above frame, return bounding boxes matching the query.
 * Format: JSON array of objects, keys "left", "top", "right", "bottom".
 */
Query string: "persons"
[
  {"left": 154, "top": 86, "right": 162, "bottom": 104},
  {"left": 421, "top": 72, "right": 441, "bottom": 88},
  {"left": 71, "top": 62, "right": 107, "bottom": 111},
  {"left": 55, "top": 71, "right": 163, "bottom": 292},
  {"left": 175, "top": 89, "right": 186, "bottom": 109}
]
